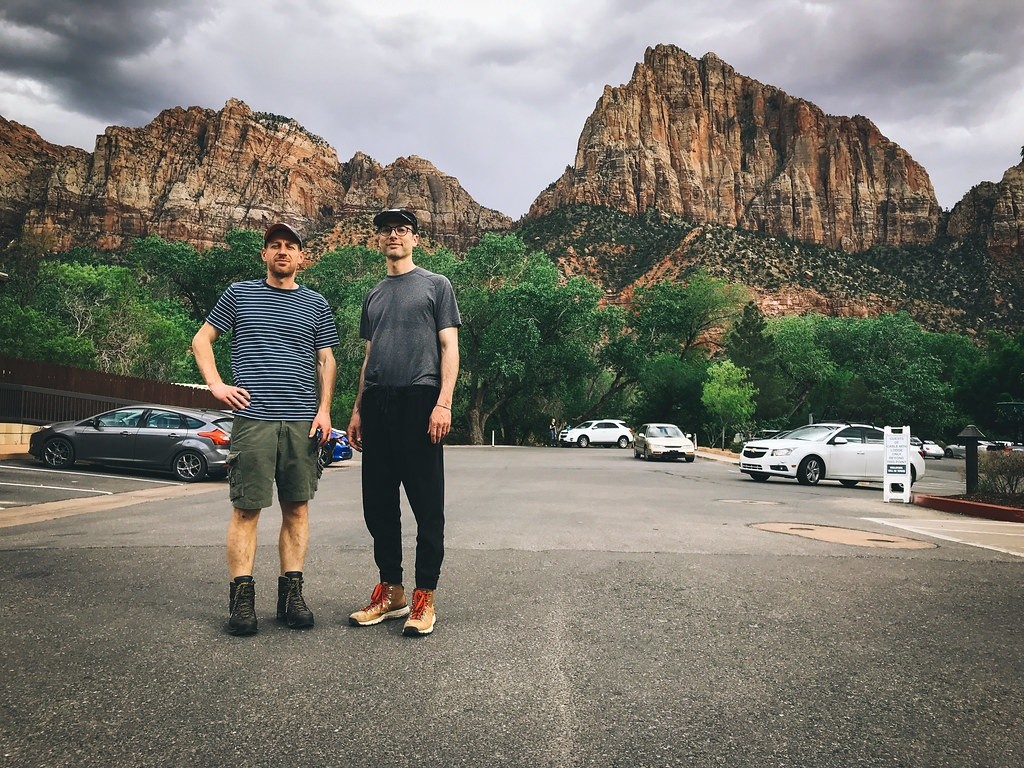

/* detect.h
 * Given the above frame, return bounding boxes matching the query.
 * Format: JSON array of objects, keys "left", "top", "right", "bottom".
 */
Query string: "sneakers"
[
  {"left": 402, "top": 588, "right": 436, "bottom": 636},
  {"left": 349, "top": 582, "right": 409, "bottom": 626},
  {"left": 226, "top": 576, "right": 258, "bottom": 635},
  {"left": 277, "top": 571, "right": 314, "bottom": 628}
]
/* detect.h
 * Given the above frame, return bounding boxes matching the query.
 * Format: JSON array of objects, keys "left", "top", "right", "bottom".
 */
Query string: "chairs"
[{"left": 157, "top": 418, "right": 167, "bottom": 428}]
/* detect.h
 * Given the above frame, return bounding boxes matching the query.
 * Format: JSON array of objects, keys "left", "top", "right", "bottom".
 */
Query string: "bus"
[{"left": 758, "top": 430, "right": 793, "bottom": 439}]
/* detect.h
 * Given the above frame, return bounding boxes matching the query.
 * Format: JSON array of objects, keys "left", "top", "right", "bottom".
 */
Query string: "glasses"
[{"left": 378, "top": 225, "right": 416, "bottom": 237}]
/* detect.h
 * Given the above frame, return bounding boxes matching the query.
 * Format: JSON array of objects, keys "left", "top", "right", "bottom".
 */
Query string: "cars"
[
  {"left": 27, "top": 404, "right": 239, "bottom": 483},
  {"left": 1017, "top": 442, "right": 1023, "bottom": 445},
  {"left": 944, "top": 444, "right": 966, "bottom": 458},
  {"left": 978, "top": 441, "right": 997, "bottom": 453},
  {"left": 922, "top": 440, "right": 945, "bottom": 459},
  {"left": 221, "top": 410, "right": 354, "bottom": 466},
  {"left": 633, "top": 423, "right": 695, "bottom": 462},
  {"left": 995, "top": 441, "right": 1014, "bottom": 450}
]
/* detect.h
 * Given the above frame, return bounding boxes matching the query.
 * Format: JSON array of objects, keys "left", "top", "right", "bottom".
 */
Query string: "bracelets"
[
  {"left": 435, "top": 404, "right": 452, "bottom": 411},
  {"left": 346, "top": 206, "right": 462, "bottom": 638}
]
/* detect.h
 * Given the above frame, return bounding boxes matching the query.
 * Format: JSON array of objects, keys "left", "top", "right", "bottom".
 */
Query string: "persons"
[{"left": 191, "top": 221, "right": 341, "bottom": 636}]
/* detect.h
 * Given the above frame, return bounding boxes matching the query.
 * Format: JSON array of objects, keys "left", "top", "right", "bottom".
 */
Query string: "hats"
[
  {"left": 373, "top": 209, "right": 417, "bottom": 233},
  {"left": 264, "top": 223, "right": 302, "bottom": 251}
]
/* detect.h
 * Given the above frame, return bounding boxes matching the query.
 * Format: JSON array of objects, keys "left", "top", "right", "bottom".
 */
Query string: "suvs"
[
  {"left": 910, "top": 435, "right": 925, "bottom": 449},
  {"left": 738, "top": 422, "right": 926, "bottom": 491},
  {"left": 558, "top": 418, "right": 636, "bottom": 449}
]
